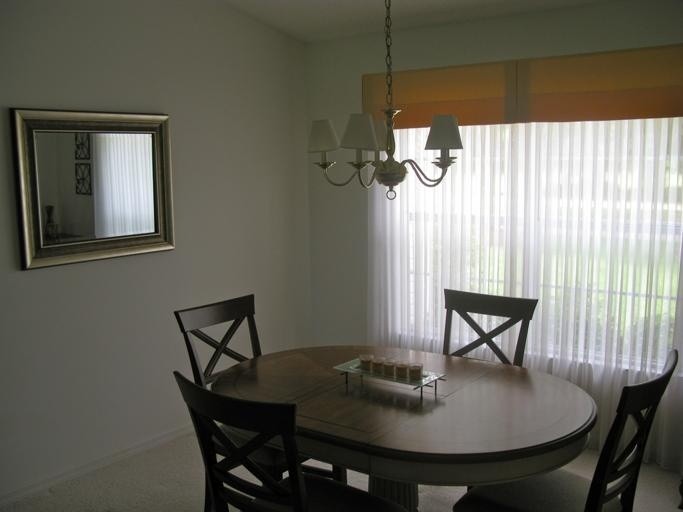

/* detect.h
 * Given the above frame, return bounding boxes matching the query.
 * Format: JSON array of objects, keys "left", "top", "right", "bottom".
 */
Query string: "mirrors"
[{"left": 9, "top": 107, "right": 176, "bottom": 270}]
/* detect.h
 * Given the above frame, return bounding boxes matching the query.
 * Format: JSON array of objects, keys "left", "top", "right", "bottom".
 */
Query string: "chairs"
[
  {"left": 453, "top": 348, "right": 679, "bottom": 510},
  {"left": 445, "top": 290, "right": 539, "bottom": 368},
  {"left": 174, "top": 370, "right": 389, "bottom": 510},
  {"left": 174, "top": 291, "right": 349, "bottom": 485}
]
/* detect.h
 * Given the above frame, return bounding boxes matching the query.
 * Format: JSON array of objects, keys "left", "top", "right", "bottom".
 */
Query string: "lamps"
[{"left": 307, "top": 0, "right": 463, "bottom": 200}]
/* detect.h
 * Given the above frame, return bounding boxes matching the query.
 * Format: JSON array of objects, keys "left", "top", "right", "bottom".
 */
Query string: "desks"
[{"left": 211, "top": 344, "right": 597, "bottom": 512}]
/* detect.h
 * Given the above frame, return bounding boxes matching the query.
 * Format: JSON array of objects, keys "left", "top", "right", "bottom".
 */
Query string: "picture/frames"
[
  {"left": 75, "top": 132, "right": 90, "bottom": 160},
  {"left": 74, "top": 163, "right": 92, "bottom": 195}
]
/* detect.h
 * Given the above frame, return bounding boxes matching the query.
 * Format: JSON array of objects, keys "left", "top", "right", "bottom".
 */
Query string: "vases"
[{"left": 45, "top": 205, "right": 57, "bottom": 241}]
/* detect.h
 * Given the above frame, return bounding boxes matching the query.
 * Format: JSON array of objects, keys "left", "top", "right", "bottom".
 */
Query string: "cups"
[{"left": 359, "top": 354, "right": 423, "bottom": 382}]
